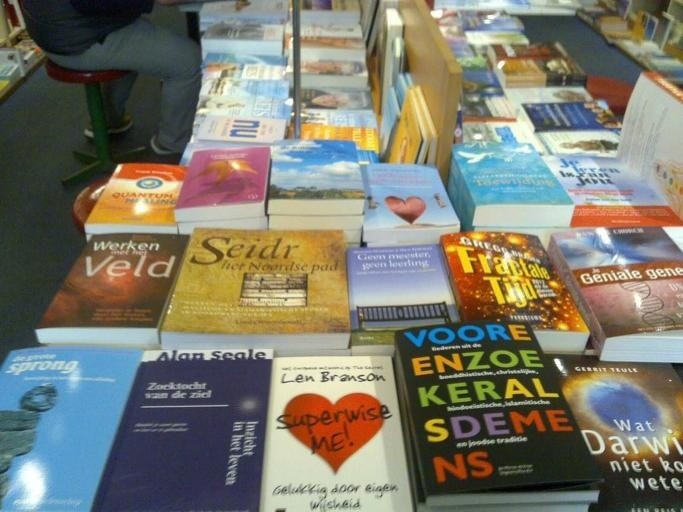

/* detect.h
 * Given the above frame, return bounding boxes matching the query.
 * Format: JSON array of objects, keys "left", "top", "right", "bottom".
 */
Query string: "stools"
[{"left": 44, "top": 57, "right": 152, "bottom": 188}]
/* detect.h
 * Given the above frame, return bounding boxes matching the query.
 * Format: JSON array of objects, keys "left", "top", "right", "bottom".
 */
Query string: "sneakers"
[
  {"left": 149, "top": 134, "right": 178, "bottom": 155},
  {"left": 83, "top": 115, "right": 133, "bottom": 139}
]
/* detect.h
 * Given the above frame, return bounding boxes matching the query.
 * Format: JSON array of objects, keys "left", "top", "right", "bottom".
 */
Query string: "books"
[
  {"left": 545, "top": 226, "right": 683, "bottom": 362},
  {"left": 559, "top": 0, "right": 682, "bottom": 85},
  {"left": 91, "top": 349, "right": 274, "bottom": 511},
  {"left": 0, "top": 350, "right": 143, "bottom": 512},
  {"left": 391, "top": 320, "right": 604, "bottom": 512},
  {"left": 285, "top": 0, "right": 380, "bottom": 156},
  {"left": 435, "top": 0, "right": 577, "bottom": 16},
  {"left": 177, "top": 0, "right": 290, "bottom": 167},
  {"left": 617, "top": 72, "right": 682, "bottom": 223},
  {"left": 35, "top": 233, "right": 187, "bottom": 344},
  {"left": 540, "top": 155, "right": 683, "bottom": 250},
  {"left": 440, "top": 232, "right": 589, "bottom": 352},
  {"left": 174, "top": 147, "right": 271, "bottom": 236},
  {"left": 360, "top": 162, "right": 461, "bottom": 247},
  {"left": 161, "top": 227, "right": 351, "bottom": 357},
  {"left": 267, "top": 140, "right": 364, "bottom": 247},
  {"left": 0, "top": 37, "right": 42, "bottom": 98},
  {"left": 544, "top": 354, "right": 683, "bottom": 511},
  {"left": 445, "top": 143, "right": 575, "bottom": 252},
  {"left": 430, "top": 10, "right": 622, "bottom": 155},
  {"left": 83, "top": 162, "right": 188, "bottom": 242},
  {"left": 259, "top": 356, "right": 413, "bottom": 512},
  {"left": 360, "top": 0, "right": 438, "bottom": 164},
  {"left": 345, "top": 244, "right": 462, "bottom": 358}
]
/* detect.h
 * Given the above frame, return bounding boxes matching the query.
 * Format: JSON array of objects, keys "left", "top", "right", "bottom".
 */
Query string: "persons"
[{"left": 17, "top": 0, "right": 201, "bottom": 156}]
